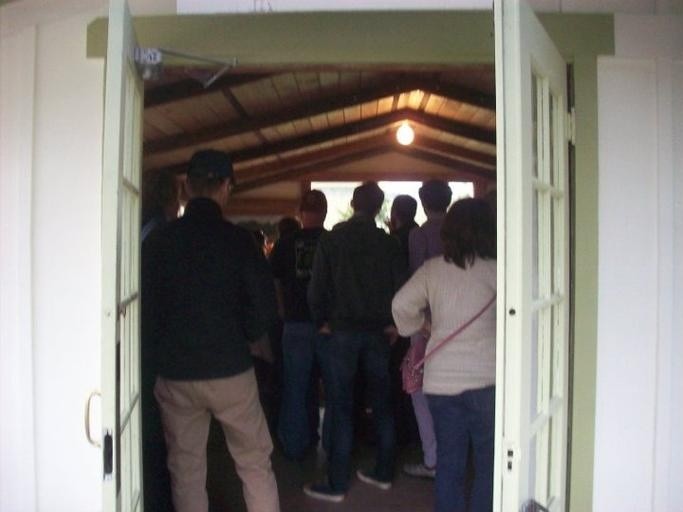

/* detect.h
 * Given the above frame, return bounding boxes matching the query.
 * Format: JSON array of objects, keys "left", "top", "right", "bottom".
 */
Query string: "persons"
[{"left": 141, "top": 149, "right": 498, "bottom": 512}]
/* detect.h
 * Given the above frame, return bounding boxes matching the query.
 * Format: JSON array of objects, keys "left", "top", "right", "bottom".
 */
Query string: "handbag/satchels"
[{"left": 402, "top": 337, "right": 426, "bottom": 393}]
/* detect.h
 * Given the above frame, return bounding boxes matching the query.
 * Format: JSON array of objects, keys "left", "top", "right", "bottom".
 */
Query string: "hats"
[{"left": 187, "top": 149, "right": 232, "bottom": 178}]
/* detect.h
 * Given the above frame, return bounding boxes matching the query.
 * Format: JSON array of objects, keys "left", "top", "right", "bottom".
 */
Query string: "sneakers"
[
  {"left": 303, "top": 481, "right": 344, "bottom": 502},
  {"left": 404, "top": 464, "right": 437, "bottom": 480},
  {"left": 357, "top": 467, "right": 392, "bottom": 489}
]
